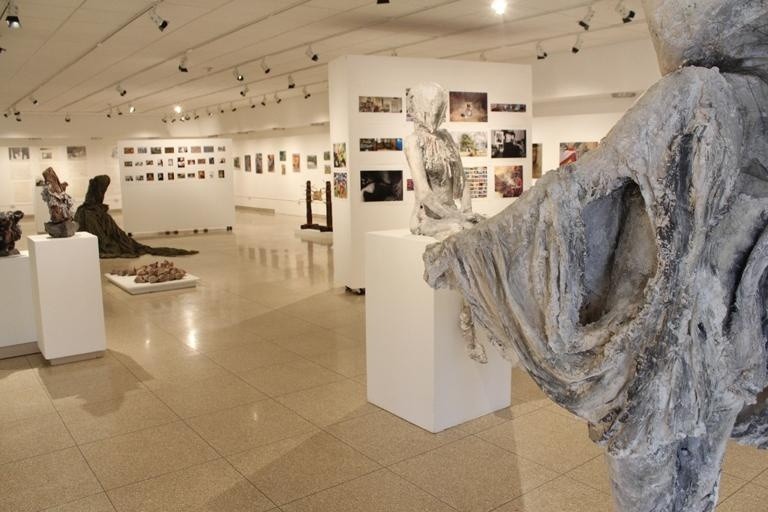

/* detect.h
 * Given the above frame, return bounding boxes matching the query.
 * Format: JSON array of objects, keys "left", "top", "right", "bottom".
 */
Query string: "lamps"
[
  {"left": 5, "top": 4, "right": 22, "bottom": 30},
  {"left": 535, "top": 0, "right": 636, "bottom": 60},
  {"left": 147, "top": 8, "right": 168, "bottom": 32},
  {"left": 233, "top": 42, "right": 319, "bottom": 97}
]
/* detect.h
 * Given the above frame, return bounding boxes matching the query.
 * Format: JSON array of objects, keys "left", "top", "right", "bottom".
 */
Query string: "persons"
[{"left": 406, "top": 86, "right": 487, "bottom": 235}]
